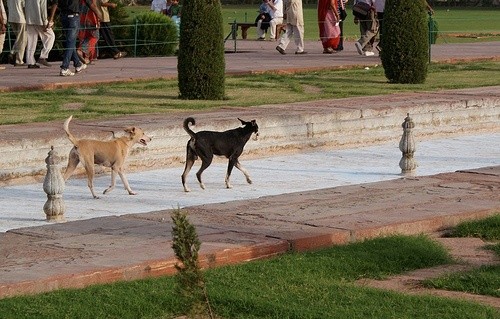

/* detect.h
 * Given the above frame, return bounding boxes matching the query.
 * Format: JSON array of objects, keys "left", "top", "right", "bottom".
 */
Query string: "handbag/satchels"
[
  {"left": 8, "top": 52, "right": 16, "bottom": 66},
  {"left": 352, "top": 2, "right": 371, "bottom": 20}
]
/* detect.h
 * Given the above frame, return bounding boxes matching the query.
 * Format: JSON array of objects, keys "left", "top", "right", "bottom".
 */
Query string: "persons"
[
  {"left": 77, "top": 0, "right": 100, "bottom": 64},
  {"left": 276, "top": 0, "right": 308, "bottom": 54},
  {"left": 6, "top": 0, "right": 28, "bottom": 66},
  {"left": 353, "top": 0, "right": 386, "bottom": 56},
  {"left": 0, "top": 0, "right": 8, "bottom": 55},
  {"left": 48, "top": 0, "right": 104, "bottom": 75},
  {"left": 255, "top": 0, "right": 284, "bottom": 41},
  {"left": 426, "top": 2, "right": 435, "bottom": 14},
  {"left": 25, "top": 0, "right": 56, "bottom": 68},
  {"left": 318, "top": 0, "right": 341, "bottom": 54},
  {"left": 93, "top": 0, "right": 127, "bottom": 60},
  {"left": 334, "top": 0, "right": 347, "bottom": 51},
  {"left": 151, "top": 0, "right": 182, "bottom": 35}
]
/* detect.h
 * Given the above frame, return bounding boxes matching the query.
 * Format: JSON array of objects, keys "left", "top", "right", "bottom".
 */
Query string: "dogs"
[
  {"left": 181, "top": 116, "right": 259, "bottom": 192},
  {"left": 61, "top": 114, "right": 151, "bottom": 199}
]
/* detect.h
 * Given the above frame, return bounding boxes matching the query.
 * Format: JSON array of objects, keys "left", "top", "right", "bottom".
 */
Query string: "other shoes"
[
  {"left": 114, "top": 52, "right": 128, "bottom": 60},
  {"left": 322, "top": 48, "right": 336, "bottom": 54},
  {"left": 269, "top": 38, "right": 275, "bottom": 42},
  {"left": 14, "top": 62, "right": 27, "bottom": 67},
  {"left": 364, "top": 51, "right": 374, "bottom": 57},
  {"left": 75, "top": 64, "right": 87, "bottom": 73},
  {"left": 28, "top": 64, "right": 41, "bottom": 69},
  {"left": 354, "top": 42, "right": 364, "bottom": 55},
  {"left": 276, "top": 46, "right": 287, "bottom": 55},
  {"left": 258, "top": 37, "right": 266, "bottom": 40},
  {"left": 60, "top": 68, "right": 75, "bottom": 76},
  {"left": 38, "top": 59, "right": 51, "bottom": 66},
  {"left": 295, "top": 51, "right": 308, "bottom": 55},
  {"left": 77, "top": 50, "right": 86, "bottom": 63}
]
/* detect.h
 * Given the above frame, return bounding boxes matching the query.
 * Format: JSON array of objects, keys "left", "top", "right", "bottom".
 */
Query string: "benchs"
[{"left": 229, "top": 22, "right": 286, "bottom": 41}]
